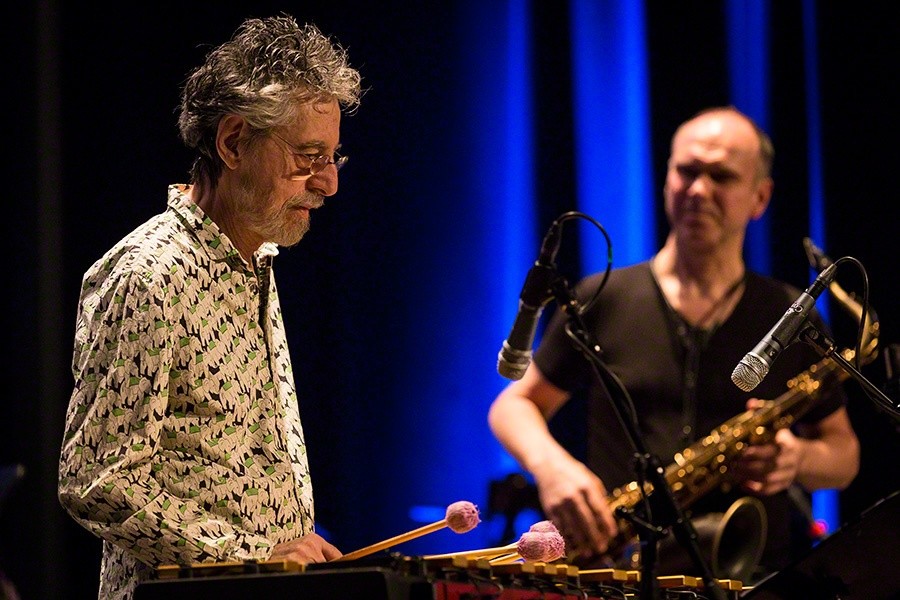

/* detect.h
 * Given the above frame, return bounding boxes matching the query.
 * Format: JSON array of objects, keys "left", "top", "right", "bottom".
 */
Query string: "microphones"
[
  {"left": 731, "top": 264, "right": 839, "bottom": 391},
  {"left": 496, "top": 220, "right": 563, "bottom": 382}
]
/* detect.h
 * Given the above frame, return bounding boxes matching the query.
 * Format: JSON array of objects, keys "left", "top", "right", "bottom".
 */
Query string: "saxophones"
[{"left": 553, "top": 235, "right": 882, "bottom": 580}]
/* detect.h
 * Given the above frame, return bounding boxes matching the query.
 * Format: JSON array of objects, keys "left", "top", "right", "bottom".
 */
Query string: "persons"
[
  {"left": 57, "top": 12, "right": 361, "bottom": 600},
  {"left": 488, "top": 104, "right": 862, "bottom": 600}
]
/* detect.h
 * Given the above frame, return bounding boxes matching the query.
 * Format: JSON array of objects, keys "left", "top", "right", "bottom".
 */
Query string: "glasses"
[{"left": 270, "top": 130, "right": 349, "bottom": 176}]
